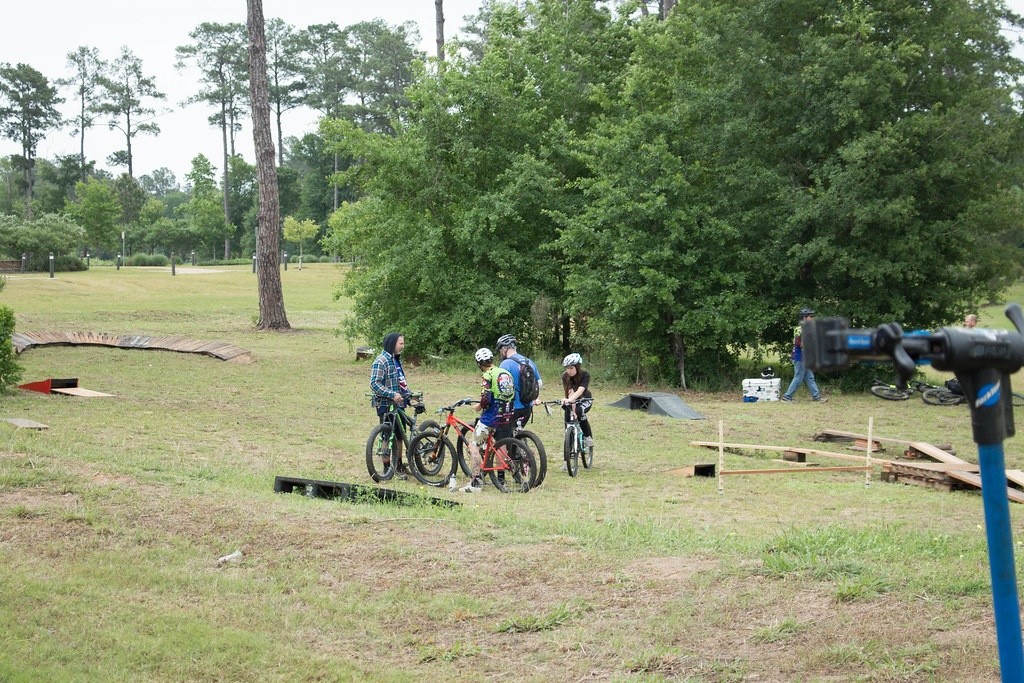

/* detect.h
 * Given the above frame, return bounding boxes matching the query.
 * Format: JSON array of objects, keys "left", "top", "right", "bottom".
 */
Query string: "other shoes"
[
  {"left": 585, "top": 436, "right": 594, "bottom": 446},
  {"left": 813, "top": 398, "right": 828, "bottom": 403},
  {"left": 397, "top": 464, "right": 411, "bottom": 474},
  {"left": 560, "top": 461, "right": 567, "bottom": 470},
  {"left": 780, "top": 397, "right": 798, "bottom": 404},
  {"left": 384, "top": 467, "right": 394, "bottom": 479}
]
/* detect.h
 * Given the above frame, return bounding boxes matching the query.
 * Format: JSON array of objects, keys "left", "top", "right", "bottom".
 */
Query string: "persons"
[
  {"left": 558, "top": 352, "right": 594, "bottom": 472},
  {"left": 495, "top": 333, "right": 542, "bottom": 460},
  {"left": 370, "top": 332, "right": 424, "bottom": 480},
  {"left": 459, "top": 347, "right": 516, "bottom": 493},
  {"left": 962, "top": 313, "right": 978, "bottom": 330},
  {"left": 781, "top": 309, "right": 828, "bottom": 403}
]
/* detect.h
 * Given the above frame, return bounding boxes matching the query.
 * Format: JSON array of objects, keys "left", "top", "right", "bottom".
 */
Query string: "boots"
[{"left": 459, "top": 475, "right": 483, "bottom": 493}]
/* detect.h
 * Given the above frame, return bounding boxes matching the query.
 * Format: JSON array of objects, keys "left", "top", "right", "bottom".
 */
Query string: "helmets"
[
  {"left": 563, "top": 353, "right": 583, "bottom": 366},
  {"left": 799, "top": 309, "right": 814, "bottom": 319},
  {"left": 495, "top": 334, "right": 518, "bottom": 350},
  {"left": 475, "top": 347, "right": 493, "bottom": 362}
]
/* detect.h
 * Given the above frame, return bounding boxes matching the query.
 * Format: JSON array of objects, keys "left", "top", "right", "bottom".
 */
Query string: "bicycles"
[
  {"left": 921, "top": 380, "right": 1024, "bottom": 407},
  {"left": 405, "top": 398, "right": 549, "bottom": 495},
  {"left": 870, "top": 377, "right": 943, "bottom": 402},
  {"left": 364, "top": 391, "right": 444, "bottom": 486},
  {"left": 540, "top": 398, "right": 595, "bottom": 478}
]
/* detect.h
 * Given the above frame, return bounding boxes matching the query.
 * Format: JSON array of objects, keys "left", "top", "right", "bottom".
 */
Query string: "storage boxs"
[{"left": 741, "top": 378, "right": 781, "bottom": 403}]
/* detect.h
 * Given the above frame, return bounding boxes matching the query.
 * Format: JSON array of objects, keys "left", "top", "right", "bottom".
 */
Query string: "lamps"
[{"left": 21, "top": 249, "right": 287, "bottom": 259}]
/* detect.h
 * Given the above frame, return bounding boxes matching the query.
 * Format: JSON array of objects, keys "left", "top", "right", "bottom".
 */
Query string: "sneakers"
[{"left": 498, "top": 479, "right": 508, "bottom": 488}]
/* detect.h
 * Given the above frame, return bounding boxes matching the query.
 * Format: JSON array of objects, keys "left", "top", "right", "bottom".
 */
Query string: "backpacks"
[{"left": 509, "top": 356, "right": 539, "bottom": 406}]
